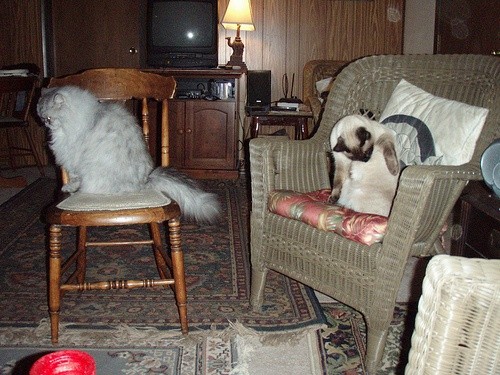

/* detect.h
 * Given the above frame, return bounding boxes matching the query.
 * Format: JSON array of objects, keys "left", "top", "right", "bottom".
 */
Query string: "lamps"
[{"left": 215, "top": 0, "right": 257, "bottom": 72}]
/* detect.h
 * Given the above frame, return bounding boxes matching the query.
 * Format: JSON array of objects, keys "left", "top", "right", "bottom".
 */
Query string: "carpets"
[
  {"left": 1, "top": 177, "right": 330, "bottom": 329},
  {"left": 0, "top": 329, "right": 243, "bottom": 375},
  {"left": 307, "top": 300, "right": 410, "bottom": 375}
]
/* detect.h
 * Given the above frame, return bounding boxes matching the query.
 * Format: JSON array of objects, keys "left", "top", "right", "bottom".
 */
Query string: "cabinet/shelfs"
[
  {"left": 455, "top": 181, "right": 500, "bottom": 256},
  {"left": 165, "top": 66, "right": 243, "bottom": 183}
]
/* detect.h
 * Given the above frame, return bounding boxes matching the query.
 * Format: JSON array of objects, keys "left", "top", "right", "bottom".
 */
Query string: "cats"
[
  {"left": 34, "top": 83, "right": 226, "bottom": 227},
  {"left": 324, "top": 106, "right": 403, "bottom": 219}
]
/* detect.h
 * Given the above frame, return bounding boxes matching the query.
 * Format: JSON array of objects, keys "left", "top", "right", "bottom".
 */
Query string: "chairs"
[
  {"left": 247, "top": 54, "right": 500, "bottom": 375},
  {"left": 301, "top": 58, "right": 351, "bottom": 131},
  {"left": 0, "top": 62, "right": 45, "bottom": 176},
  {"left": 47, "top": 66, "right": 190, "bottom": 345},
  {"left": 404, "top": 256, "right": 500, "bottom": 375}
]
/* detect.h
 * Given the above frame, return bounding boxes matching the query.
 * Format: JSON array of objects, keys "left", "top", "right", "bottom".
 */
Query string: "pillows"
[{"left": 377, "top": 76, "right": 490, "bottom": 171}]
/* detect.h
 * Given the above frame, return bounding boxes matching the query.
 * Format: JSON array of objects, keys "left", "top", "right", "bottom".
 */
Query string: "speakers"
[{"left": 247, "top": 70, "right": 271, "bottom": 112}]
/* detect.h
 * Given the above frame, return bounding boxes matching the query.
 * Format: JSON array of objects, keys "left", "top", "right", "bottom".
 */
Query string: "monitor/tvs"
[{"left": 140, "top": 0, "right": 219, "bottom": 69}]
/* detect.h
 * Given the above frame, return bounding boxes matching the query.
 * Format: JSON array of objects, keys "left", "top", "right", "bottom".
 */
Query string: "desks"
[
  {"left": 0, "top": 67, "right": 38, "bottom": 128},
  {"left": 248, "top": 109, "right": 314, "bottom": 141}
]
